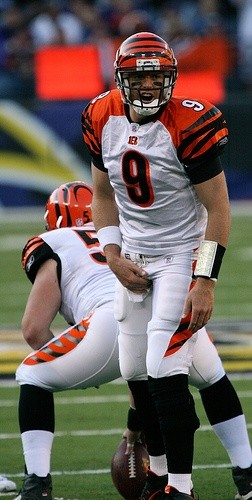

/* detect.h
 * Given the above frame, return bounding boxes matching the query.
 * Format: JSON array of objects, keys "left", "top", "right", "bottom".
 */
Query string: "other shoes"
[
  {"left": 12, "top": 465, "right": 52, "bottom": 500},
  {"left": 232, "top": 464, "right": 252, "bottom": 500},
  {"left": 140, "top": 466, "right": 168, "bottom": 500},
  {"left": 0, "top": 477, "right": 16, "bottom": 491},
  {"left": 146, "top": 485, "right": 195, "bottom": 500}
]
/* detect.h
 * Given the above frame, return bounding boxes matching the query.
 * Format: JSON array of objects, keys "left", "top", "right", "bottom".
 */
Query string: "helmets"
[
  {"left": 44, "top": 181, "right": 95, "bottom": 232},
  {"left": 112, "top": 32, "right": 178, "bottom": 115}
]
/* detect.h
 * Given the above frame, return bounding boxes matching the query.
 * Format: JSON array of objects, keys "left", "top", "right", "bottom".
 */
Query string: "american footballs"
[{"left": 110, "top": 438, "right": 150, "bottom": 500}]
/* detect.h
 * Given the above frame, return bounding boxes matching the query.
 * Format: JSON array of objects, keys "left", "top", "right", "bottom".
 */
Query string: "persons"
[
  {"left": 9, "top": 180, "right": 252, "bottom": 500},
  {"left": 79, "top": 30, "right": 233, "bottom": 500}
]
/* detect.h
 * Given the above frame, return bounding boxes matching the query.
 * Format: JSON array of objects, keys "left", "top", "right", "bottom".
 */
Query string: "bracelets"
[{"left": 97, "top": 224, "right": 124, "bottom": 251}]
